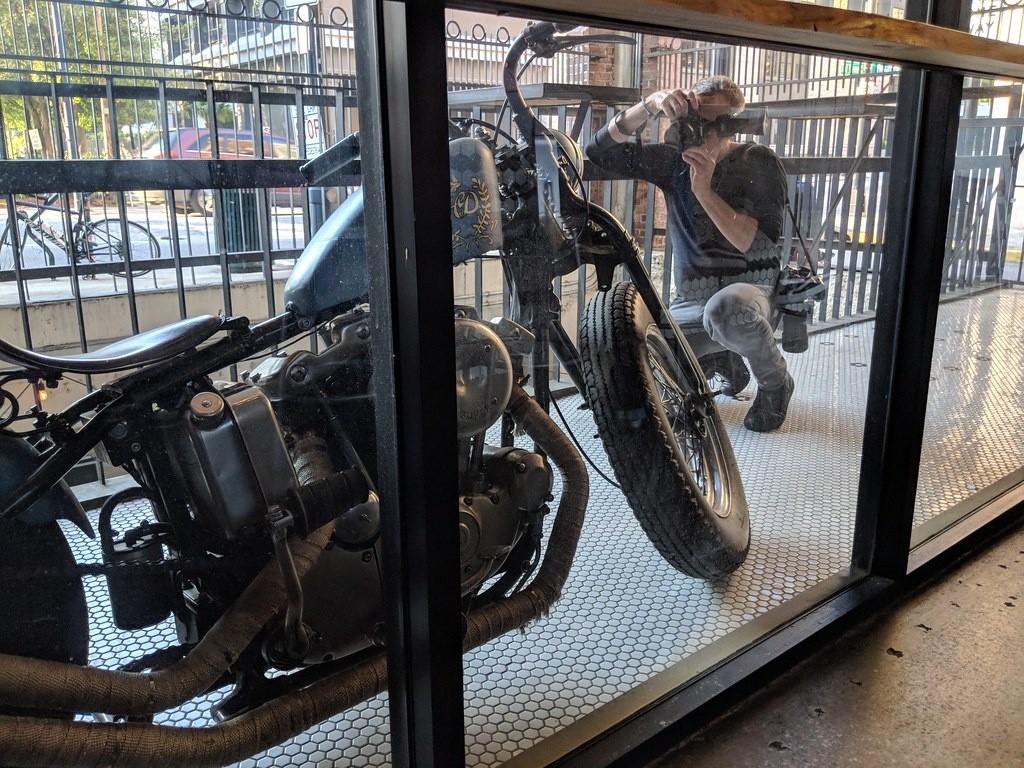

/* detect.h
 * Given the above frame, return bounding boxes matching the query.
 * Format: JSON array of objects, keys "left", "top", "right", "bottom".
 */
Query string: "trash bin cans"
[
  {"left": 308, "top": 189, "right": 330, "bottom": 239},
  {"left": 212, "top": 188, "right": 274, "bottom": 273}
]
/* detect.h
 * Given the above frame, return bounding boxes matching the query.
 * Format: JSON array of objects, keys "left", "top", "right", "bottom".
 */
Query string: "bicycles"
[{"left": 0, "top": 192, "right": 161, "bottom": 280}]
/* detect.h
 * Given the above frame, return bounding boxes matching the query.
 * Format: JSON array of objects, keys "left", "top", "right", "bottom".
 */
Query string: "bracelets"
[{"left": 641, "top": 98, "right": 661, "bottom": 118}]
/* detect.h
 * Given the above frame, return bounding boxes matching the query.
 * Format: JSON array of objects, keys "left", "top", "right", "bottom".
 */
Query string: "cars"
[{"left": 124, "top": 127, "right": 308, "bottom": 216}]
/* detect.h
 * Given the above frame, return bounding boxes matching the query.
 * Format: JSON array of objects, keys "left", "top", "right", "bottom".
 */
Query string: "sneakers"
[
  {"left": 710, "top": 351, "right": 749, "bottom": 397},
  {"left": 744, "top": 372, "right": 795, "bottom": 431}
]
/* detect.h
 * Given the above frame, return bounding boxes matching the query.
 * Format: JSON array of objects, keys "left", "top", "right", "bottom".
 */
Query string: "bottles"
[{"left": 37, "top": 220, "right": 62, "bottom": 240}]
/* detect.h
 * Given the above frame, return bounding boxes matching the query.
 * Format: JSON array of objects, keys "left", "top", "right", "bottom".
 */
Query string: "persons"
[{"left": 586, "top": 76, "right": 795, "bottom": 434}]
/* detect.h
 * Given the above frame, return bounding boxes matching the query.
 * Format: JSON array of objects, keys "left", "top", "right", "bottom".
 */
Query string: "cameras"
[
  {"left": 663, "top": 97, "right": 767, "bottom": 154},
  {"left": 774, "top": 265, "right": 825, "bottom": 353}
]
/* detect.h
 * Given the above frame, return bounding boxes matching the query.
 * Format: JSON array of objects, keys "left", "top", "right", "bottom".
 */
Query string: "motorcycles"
[{"left": 0, "top": 18, "right": 751, "bottom": 767}]
